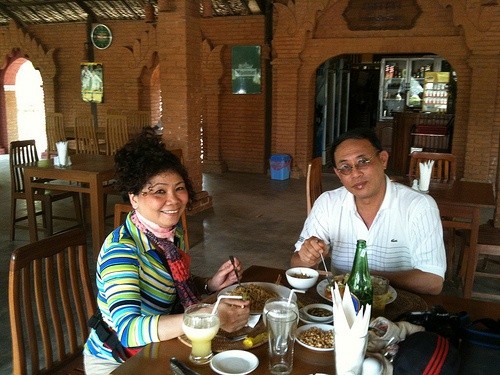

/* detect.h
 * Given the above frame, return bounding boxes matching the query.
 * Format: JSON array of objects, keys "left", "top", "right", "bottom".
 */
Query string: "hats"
[{"left": 393, "top": 331, "right": 467, "bottom": 375}]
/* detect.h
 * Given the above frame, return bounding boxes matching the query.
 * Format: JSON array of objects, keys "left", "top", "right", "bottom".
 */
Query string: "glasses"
[{"left": 335, "top": 149, "right": 380, "bottom": 176}]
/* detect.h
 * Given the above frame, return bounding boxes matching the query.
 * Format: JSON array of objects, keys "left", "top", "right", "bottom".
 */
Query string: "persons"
[
  {"left": 82, "top": 129, "right": 251, "bottom": 375},
  {"left": 290, "top": 128, "right": 447, "bottom": 296}
]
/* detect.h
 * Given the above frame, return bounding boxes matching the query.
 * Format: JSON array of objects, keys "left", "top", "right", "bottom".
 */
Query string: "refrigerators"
[{"left": 421, "top": 71, "right": 456, "bottom": 114}]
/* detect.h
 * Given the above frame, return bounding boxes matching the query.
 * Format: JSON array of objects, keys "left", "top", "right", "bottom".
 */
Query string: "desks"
[
  {"left": 14, "top": 154, "right": 117, "bottom": 261},
  {"left": 390, "top": 176, "right": 496, "bottom": 209},
  {"left": 63, "top": 126, "right": 163, "bottom": 139}
]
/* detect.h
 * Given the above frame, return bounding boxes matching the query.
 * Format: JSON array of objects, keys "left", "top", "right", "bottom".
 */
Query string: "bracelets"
[{"left": 204, "top": 280, "right": 216, "bottom": 296}]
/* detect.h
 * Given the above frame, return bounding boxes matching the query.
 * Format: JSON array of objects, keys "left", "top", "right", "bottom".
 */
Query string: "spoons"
[
  {"left": 320, "top": 253, "right": 335, "bottom": 289},
  {"left": 229, "top": 256, "right": 245, "bottom": 290}
]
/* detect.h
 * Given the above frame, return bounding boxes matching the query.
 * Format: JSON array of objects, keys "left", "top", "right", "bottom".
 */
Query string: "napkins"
[
  {"left": 245, "top": 314, "right": 261, "bottom": 328},
  {"left": 418, "top": 160, "right": 435, "bottom": 190},
  {"left": 330, "top": 281, "right": 371, "bottom": 375},
  {"left": 56, "top": 141, "right": 69, "bottom": 164}
]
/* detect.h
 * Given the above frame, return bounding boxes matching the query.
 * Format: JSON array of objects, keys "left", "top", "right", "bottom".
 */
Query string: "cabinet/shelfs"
[
  {"left": 422, "top": 72, "right": 456, "bottom": 114},
  {"left": 376, "top": 58, "right": 457, "bottom": 169}
]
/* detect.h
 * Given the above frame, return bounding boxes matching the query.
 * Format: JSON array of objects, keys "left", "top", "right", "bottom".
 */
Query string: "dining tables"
[{"left": 111, "top": 266, "right": 500, "bottom": 375}]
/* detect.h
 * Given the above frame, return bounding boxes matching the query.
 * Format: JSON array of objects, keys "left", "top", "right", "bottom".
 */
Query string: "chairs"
[
  {"left": 114, "top": 150, "right": 191, "bottom": 253},
  {"left": 45, "top": 109, "right": 151, "bottom": 159},
  {"left": 437, "top": 205, "right": 479, "bottom": 299},
  {"left": 456, "top": 151, "right": 500, "bottom": 302},
  {"left": 10, "top": 140, "right": 83, "bottom": 243},
  {"left": 409, "top": 151, "right": 457, "bottom": 178},
  {"left": 8, "top": 226, "right": 96, "bottom": 375},
  {"left": 306, "top": 158, "right": 322, "bottom": 219}
]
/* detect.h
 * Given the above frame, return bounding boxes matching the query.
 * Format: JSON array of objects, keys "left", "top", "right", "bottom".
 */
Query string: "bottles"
[
  {"left": 412, "top": 179, "right": 418, "bottom": 191},
  {"left": 346, "top": 240, "right": 373, "bottom": 321}
]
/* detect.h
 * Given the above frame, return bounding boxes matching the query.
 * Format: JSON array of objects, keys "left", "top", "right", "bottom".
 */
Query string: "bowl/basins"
[
  {"left": 286, "top": 267, "right": 319, "bottom": 289},
  {"left": 303, "top": 304, "right": 333, "bottom": 321}
]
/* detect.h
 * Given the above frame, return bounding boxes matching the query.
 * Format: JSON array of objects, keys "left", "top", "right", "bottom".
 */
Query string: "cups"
[
  {"left": 182, "top": 303, "right": 220, "bottom": 365},
  {"left": 58, "top": 154, "right": 67, "bottom": 168},
  {"left": 262, "top": 296, "right": 298, "bottom": 375},
  {"left": 420, "top": 176, "right": 430, "bottom": 193},
  {"left": 370, "top": 275, "right": 389, "bottom": 318}
]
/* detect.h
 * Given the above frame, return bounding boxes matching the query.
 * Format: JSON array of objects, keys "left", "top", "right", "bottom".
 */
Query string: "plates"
[
  {"left": 210, "top": 350, "right": 259, "bottom": 375},
  {"left": 294, "top": 324, "right": 334, "bottom": 351},
  {"left": 298, "top": 308, "right": 334, "bottom": 324},
  {"left": 316, "top": 275, "right": 397, "bottom": 308},
  {"left": 217, "top": 282, "right": 297, "bottom": 315}
]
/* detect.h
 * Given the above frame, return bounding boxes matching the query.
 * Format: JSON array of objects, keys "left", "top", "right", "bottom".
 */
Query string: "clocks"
[{"left": 91, "top": 23, "right": 113, "bottom": 50}]
